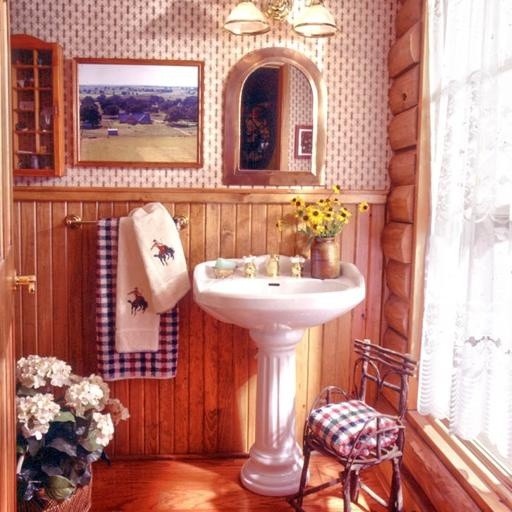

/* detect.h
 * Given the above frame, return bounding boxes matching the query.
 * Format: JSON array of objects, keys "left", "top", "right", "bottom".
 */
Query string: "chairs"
[{"left": 296, "top": 339, "right": 416, "bottom": 512}]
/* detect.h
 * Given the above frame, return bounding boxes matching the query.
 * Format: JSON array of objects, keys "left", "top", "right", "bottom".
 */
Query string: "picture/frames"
[
  {"left": 294, "top": 125, "right": 313, "bottom": 159},
  {"left": 69, "top": 57, "right": 205, "bottom": 168}
]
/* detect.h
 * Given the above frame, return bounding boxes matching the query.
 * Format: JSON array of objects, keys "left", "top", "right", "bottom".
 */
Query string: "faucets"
[{"left": 266, "top": 251, "right": 280, "bottom": 277}]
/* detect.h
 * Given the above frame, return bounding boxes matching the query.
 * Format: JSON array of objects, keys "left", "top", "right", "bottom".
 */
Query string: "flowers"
[
  {"left": 275, "top": 184, "right": 370, "bottom": 238},
  {"left": 16, "top": 354, "right": 131, "bottom": 501}
]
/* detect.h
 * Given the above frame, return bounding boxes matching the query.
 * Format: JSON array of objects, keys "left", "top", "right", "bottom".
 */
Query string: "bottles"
[
  {"left": 266, "top": 254, "right": 280, "bottom": 276},
  {"left": 18, "top": 155, "right": 50, "bottom": 170}
]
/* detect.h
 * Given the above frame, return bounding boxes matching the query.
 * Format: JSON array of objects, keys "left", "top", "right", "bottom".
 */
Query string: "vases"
[
  {"left": 18, "top": 463, "right": 92, "bottom": 512},
  {"left": 310, "top": 238, "right": 341, "bottom": 279}
]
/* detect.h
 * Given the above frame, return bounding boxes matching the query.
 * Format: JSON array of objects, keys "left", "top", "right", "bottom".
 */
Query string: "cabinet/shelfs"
[{"left": 10, "top": 34, "right": 66, "bottom": 177}]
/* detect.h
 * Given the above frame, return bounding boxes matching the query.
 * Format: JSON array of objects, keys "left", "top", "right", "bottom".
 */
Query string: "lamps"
[{"left": 224, "top": 0, "right": 336, "bottom": 38}]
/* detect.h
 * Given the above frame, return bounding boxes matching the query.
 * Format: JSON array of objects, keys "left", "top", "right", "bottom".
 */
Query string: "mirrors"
[{"left": 240, "top": 61, "right": 314, "bottom": 174}]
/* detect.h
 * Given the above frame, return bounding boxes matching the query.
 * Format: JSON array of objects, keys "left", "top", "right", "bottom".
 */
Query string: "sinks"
[{"left": 194, "top": 253, "right": 366, "bottom": 334}]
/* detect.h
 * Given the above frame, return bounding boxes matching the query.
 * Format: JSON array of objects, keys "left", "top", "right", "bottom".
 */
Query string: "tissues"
[{"left": 213, "top": 256, "right": 240, "bottom": 280}]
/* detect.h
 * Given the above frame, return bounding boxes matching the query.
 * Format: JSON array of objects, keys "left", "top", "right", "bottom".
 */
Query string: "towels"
[{"left": 96, "top": 201, "right": 192, "bottom": 382}]
